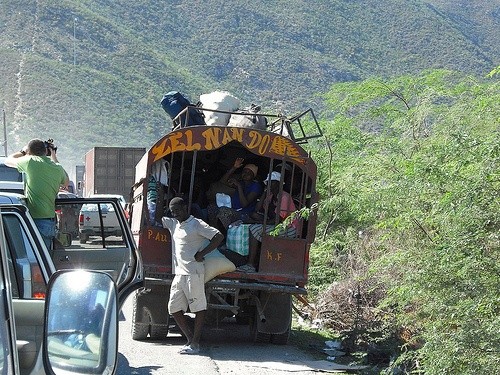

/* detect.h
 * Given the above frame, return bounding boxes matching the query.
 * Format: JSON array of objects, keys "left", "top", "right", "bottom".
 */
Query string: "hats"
[
  {"left": 244, "top": 164, "right": 258, "bottom": 177},
  {"left": 264, "top": 171, "right": 285, "bottom": 185},
  {"left": 276, "top": 163, "right": 292, "bottom": 171}
]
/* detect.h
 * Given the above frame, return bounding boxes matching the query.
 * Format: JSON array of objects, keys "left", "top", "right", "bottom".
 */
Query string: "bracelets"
[{"left": 20, "top": 149, "right": 26, "bottom": 155}]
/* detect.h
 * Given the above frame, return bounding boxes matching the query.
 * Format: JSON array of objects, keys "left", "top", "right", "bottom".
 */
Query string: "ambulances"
[{"left": 79, "top": 193, "right": 126, "bottom": 244}]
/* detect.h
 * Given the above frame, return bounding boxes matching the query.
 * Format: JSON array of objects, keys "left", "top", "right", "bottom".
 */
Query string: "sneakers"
[{"left": 236, "top": 264, "right": 256, "bottom": 273}]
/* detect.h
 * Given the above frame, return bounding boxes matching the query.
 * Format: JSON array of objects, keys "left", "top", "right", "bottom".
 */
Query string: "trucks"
[
  {"left": 133, "top": 105, "right": 318, "bottom": 346},
  {"left": 68, "top": 145, "right": 146, "bottom": 204}
]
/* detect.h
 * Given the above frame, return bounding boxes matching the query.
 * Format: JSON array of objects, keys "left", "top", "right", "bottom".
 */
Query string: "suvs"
[
  {"left": 0, "top": 156, "right": 27, "bottom": 193},
  {"left": 55, "top": 205, "right": 82, "bottom": 240}
]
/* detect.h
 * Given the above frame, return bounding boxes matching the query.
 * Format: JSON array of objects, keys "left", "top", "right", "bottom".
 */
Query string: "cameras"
[{"left": 44, "top": 138, "right": 57, "bottom": 156}]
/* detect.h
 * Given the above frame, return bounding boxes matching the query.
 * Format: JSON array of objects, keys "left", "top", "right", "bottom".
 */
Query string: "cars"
[
  {"left": 1, "top": 204, "right": 120, "bottom": 373},
  {"left": 0, "top": 193, "right": 146, "bottom": 322}
]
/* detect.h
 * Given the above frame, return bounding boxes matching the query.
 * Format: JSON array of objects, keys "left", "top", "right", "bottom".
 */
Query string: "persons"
[
  {"left": 147, "top": 157, "right": 302, "bottom": 274},
  {"left": 154, "top": 180, "right": 223, "bottom": 354},
  {"left": 3, "top": 138, "right": 70, "bottom": 286}
]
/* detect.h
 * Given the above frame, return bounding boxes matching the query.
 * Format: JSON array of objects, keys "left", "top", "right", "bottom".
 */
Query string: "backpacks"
[{"left": 161, "top": 91, "right": 207, "bottom": 127}]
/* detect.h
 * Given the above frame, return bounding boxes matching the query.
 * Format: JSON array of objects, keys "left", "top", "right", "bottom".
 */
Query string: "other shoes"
[{"left": 177, "top": 345, "right": 200, "bottom": 354}]
